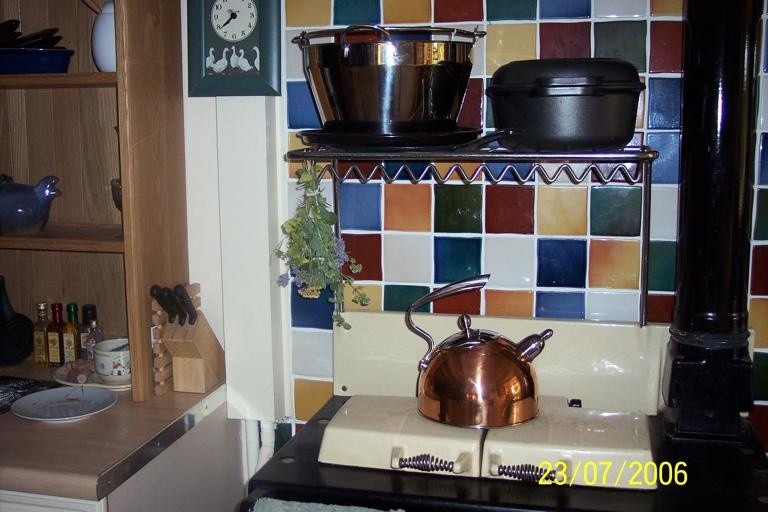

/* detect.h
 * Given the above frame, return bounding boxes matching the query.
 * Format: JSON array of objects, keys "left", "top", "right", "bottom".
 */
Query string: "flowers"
[{"left": 273, "top": 159, "right": 372, "bottom": 334}]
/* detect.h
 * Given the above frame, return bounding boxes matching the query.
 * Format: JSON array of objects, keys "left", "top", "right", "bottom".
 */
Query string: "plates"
[
  {"left": 52, "top": 359, "right": 132, "bottom": 392},
  {"left": 10, "top": 384, "right": 118, "bottom": 425}
]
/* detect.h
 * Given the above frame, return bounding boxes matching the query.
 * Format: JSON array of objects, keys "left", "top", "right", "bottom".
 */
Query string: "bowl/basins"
[
  {"left": 0, "top": 46, "right": 75, "bottom": 75},
  {"left": 291, "top": 22, "right": 488, "bottom": 129},
  {"left": 92, "top": 337, "right": 132, "bottom": 386}
]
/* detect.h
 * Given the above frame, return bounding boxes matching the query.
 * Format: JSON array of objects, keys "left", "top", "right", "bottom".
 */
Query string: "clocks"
[{"left": 185, "top": 0, "right": 285, "bottom": 98}]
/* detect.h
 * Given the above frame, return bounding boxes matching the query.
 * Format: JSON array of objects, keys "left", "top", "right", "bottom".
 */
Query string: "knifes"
[{"left": 149, "top": 284, "right": 198, "bottom": 326}]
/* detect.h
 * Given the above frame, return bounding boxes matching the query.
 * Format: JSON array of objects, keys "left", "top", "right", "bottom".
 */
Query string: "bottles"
[
  {"left": 79, "top": 303, "right": 103, "bottom": 362},
  {"left": 46, "top": 302, "right": 65, "bottom": 368},
  {"left": 32, "top": 301, "right": 52, "bottom": 369},
  {"left": 61, "top": 302, "right": 81, "bottom": 365}
]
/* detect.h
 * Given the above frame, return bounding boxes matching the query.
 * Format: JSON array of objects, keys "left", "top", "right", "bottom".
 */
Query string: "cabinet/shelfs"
[{"left": 0, "top": 1, "right": 248, "bottom": 511}]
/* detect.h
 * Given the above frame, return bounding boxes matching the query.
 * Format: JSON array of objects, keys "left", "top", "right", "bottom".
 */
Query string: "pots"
[
  {"left": 294, "top": 124, "right": 517, "bottom": 154},
  {"left": 483, "top": 57, "right": 646, "bottom": 155}
]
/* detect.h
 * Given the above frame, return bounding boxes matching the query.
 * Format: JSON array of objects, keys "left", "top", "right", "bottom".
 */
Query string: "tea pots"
[{"left": 404, "top": 273, "right": 555, "bottom": 428}]
